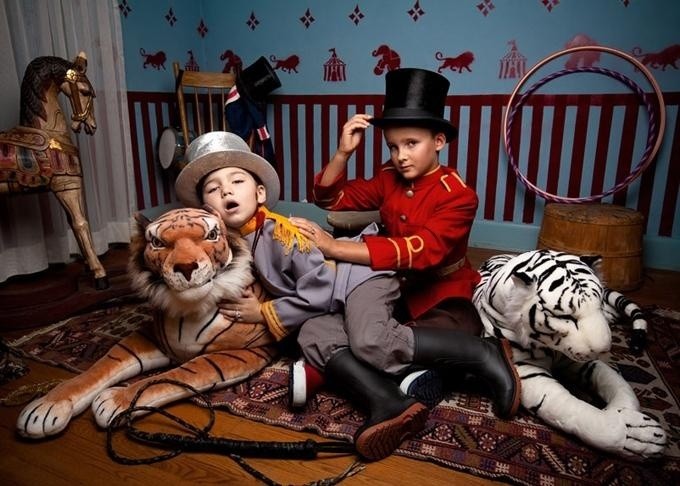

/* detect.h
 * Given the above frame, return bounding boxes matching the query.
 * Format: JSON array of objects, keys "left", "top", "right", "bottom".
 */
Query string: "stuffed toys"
[
  {"left": 11, "top": 204, "right": 293, "bottom": 445},
  {"left": 466, "top": 243, "right": 669, "bottom": 467}
]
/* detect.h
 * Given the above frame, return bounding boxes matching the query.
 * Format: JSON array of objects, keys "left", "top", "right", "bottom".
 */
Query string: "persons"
[
  {"left": 194, "top": 163, "right": 525, "bottom": 464},
  {"left": 285, "top": 112, "right": 486, "bottom": 409}
]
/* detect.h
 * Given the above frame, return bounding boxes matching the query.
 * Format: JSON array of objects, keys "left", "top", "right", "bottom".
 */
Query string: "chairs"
[{"left": 174, "top": 60, "right": 254, "bottom": 152}]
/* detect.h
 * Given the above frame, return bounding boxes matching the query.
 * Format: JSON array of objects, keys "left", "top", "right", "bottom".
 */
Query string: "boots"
[
  {"left": 324, "top": 345, "right": 431, "bottom": 460},
  {"left": 410, "top": 326, "right": 521, "bottom": 420}
]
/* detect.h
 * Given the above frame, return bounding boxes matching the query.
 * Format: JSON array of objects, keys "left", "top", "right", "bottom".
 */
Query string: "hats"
[
  {"left": 235, "top": 56, "right": 281, "bottom": 110},
  {"left": 367, "top": 67, "right": 458, "bottom": 143},
  {"left": 174, "top": 130, "right": 281, "bottom": 211}
]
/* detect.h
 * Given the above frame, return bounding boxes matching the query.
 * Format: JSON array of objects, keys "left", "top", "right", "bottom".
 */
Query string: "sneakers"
[
  {"left": 289, "top": 354, "right": 326, "bottom": 407},
  {"left": 400, "top": 366, "right": 449, "bottom": 410}
]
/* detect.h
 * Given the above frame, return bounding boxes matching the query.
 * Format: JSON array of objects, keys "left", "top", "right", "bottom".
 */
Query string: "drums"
[{"left": 156, "top": 126, "right": 196, "bottom": 170}]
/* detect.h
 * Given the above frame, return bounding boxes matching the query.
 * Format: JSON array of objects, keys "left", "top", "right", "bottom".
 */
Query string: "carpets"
[{"left": 9, "top": 291, "right": 680, "bottom": 486}]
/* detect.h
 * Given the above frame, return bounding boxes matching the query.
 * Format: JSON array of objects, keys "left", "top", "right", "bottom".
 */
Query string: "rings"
[
  {"left": 312, "top": 229, "right": 316, "bottom": 235},
  {"left": 234, "top": 310, "right": 241, "bottom": 320}
]
